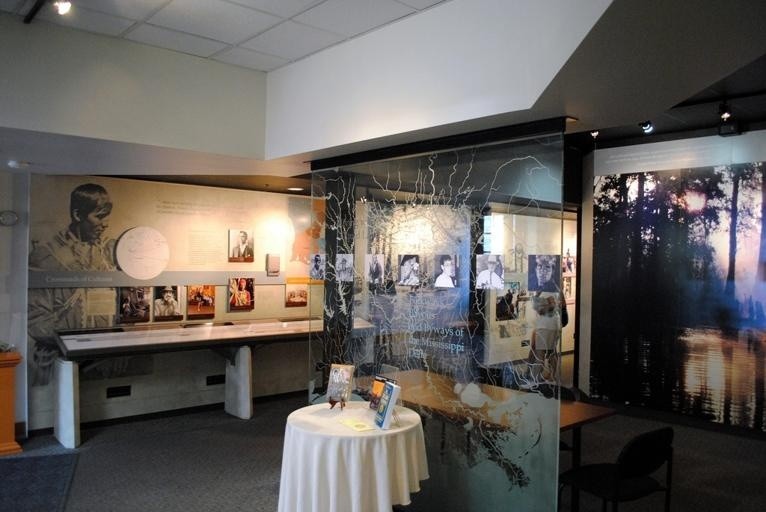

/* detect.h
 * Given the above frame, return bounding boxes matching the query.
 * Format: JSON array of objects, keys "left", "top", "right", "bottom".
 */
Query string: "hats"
[{"left": 162, "top": 286, "right": 176, "bottom": 292}]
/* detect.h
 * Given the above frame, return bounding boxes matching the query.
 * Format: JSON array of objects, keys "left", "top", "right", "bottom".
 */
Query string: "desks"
[
  {"left": 354, "top": 369, "right": 622, "bottom": 512},
  {"left": 286, "top": 400, "right": 423, "bottom": 512}
]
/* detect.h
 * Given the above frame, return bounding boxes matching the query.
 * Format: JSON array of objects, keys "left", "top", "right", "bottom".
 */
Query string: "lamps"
[
  {"left": 639, "top": 120, "right": 655, "bottom": 136},
  {"left": 717, "top": 91, "right": 732, "bottom": 123}
]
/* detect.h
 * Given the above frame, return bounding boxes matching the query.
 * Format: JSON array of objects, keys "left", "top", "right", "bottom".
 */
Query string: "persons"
[
  {"left": 196, "top": 287, "right": 206, "bottom": 312},
  {"left": 523, "top": 291, "right": 568, "bottom": 383},
  {"left": 231, "top": 230, "right": 253, "bottom": 257},
  {"left": 311, "top": 255, "right": 324, "bottom": 279},
  {"left": 27, "top": 183, "right": 130, "bottom": 386},
  {"left": 435, "top": 255, "right": 456, "bottom": 287},
  {"left": 475, "top": 255, "right": 503, "bottom": 290},
  {"left": 230, "top": 279, "right": 251, "bottom": 306},
  {"left": 533, "top": 256, "right": 560, "bottom": 291},
  {"left": 401, "top": 255, "right": 419, "bottom": 285},
  {"left": 122, "top": 288, "right": 150, "bottom": 318},
  {"left": 368, "top": 255, "right": 383, "bottom": 283},
  {"left": 154, "top": 286, "right": 177, "bottom": 316},
  {"left": 337, "top": 259, "right": 352, "bottom": 280}
]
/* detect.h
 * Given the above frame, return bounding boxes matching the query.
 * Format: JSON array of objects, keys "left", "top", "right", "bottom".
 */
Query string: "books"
[
  {"left": 370, "top": 373, "right": 397, "bottom": 412},
  {"left": 326, "top": 363, "right": 355, "bottom": 402},
  {"left": 373, "top": 381, "right": 402, "bottom": 431}
]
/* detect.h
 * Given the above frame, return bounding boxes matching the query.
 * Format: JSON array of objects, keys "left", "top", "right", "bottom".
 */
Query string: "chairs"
[
  {"left": 439, "top": 368, "right": 576, "bottom": 464},
  {"left": 558, "top": 425, "right": 676, "bottom": 511}
]
